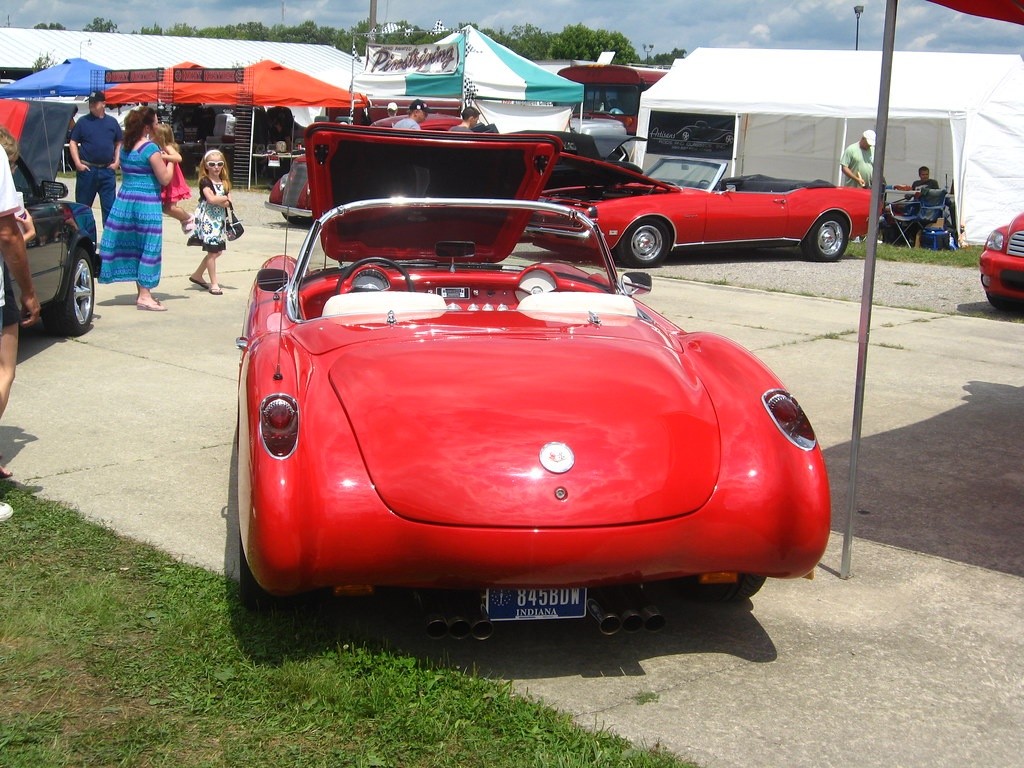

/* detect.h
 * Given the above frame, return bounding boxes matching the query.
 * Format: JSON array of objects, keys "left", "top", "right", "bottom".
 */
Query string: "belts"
[{"left": 80, "top": 159, "right": 110, "bottom": 167}]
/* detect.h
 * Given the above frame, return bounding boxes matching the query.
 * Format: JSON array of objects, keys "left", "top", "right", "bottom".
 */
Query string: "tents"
[
  {"left": 352, "top": 24, "right": 584, "bottom": 134},
  {"left": 0, "top": 57, "right": 117, "bottom": 99},
  {"left": 101, "top": 58, "right": 367, "bottom": 191},
  {"left": 633, "top": 48, "right": 1024, "bottom": 248}
]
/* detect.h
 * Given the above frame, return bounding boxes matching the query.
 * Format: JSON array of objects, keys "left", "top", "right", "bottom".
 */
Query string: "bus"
[{"left": 549, "top": 63, "right": 673, "bottom": 132}]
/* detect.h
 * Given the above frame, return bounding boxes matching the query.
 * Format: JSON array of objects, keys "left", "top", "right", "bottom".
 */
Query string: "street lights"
[
  {"left": 78, "top": 38, "right": 92, "bottom": 58},
  {"left": 642, "top": 43, "right": 655, "bottom": 66},
  {"left": 852, "top": 4, "right": 864, "bottom": 52}
]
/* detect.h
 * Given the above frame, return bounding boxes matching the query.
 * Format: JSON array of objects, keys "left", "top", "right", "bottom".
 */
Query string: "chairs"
[
  {"left": 884, "top": 189, "right": 948, "bottom": 248},
  {"left": 321, "top": 292, "right": 447, "bottom": 318},
  {"left": 517, "top": 292, "right": 638, "bottom": 318}
]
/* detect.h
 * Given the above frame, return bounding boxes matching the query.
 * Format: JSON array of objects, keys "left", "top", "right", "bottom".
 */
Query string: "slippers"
[
  {"left": 189, "top": 276, "right": 209, "bottom": 289},
  {"left": 209, "top": 289, "right": 223, "bottom": 295}
]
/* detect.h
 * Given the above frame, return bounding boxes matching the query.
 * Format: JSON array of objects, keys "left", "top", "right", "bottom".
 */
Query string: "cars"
[
  {"left": 8, "top": 152, "right": 102, "bottom": 339},
  {"left": 980, "top": 214, "right": 1024, "bottom": 313},
  {"left": 264, "top": 113, "right": 464, "bottom": 228}
]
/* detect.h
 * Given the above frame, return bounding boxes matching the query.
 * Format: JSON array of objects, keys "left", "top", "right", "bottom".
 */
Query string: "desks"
[
  {"left": 253, "top": 153, "right": 301, "bottom": 186},
  {"left": 884, "top": 189, "right": 920, "bottom": 244},
  {"left": 205, "top": 143, "right": 234, "bottom": 152}
]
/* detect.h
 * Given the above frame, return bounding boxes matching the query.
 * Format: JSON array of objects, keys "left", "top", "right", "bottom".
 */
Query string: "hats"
[
  {"left": 388, "top": 101, "right": 398, "bottom": 110},
  {"left": 863, "top": 130, "right": 876, "bottom": 145},
  {"left": 409, "top": 98, "right": 432, "bottom": 112},
  {"left": 84, "top": 90, "right": 106, "bottom": 102}
]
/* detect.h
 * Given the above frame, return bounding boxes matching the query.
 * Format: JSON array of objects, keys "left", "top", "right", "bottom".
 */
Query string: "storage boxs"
[{"left": 920, "top": 227, "right": 950, "bottom": 251}]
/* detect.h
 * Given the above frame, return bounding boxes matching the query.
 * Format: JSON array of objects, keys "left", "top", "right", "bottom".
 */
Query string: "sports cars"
[
  {"left": 221, "top": 117, "right": 835, "bottom": 633},
  {"left": 524, "top": 133, "right": 883, "bottom": 271}
]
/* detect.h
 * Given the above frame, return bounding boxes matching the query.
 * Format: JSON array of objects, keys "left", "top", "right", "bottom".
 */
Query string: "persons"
[
  {"left": 840, "top": 130, "right": 883, "bottom": 244},
  {"left": 912, "top": 166, "right": 939, "bottom": 191},
  {"left": 186, "top": 149, "right": 232, "bottom": 294},
  {"left": 449, "top": 106, "right": 480, "bottom": 132},
  {"left": 387, "top": 102, "right": 398, "bottom": 117},
  {"left": 393, "top": 99, "right": 431, "bottom": 129},
  {"left": 69, "top": 93, "right": 124, "bottom": 228},
  {"left": 98, "top": 106, "right": 174, "bottom": 311},
  {"left": 149, "top": 123, "right": 196, "bottom": 234},
  {"left": 0, "top": 125, "right": 42, "bottom": 523}
]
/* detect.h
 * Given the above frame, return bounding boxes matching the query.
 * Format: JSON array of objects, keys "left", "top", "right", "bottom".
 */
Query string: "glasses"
[{"left": 206, "top": 160, "right": 225, "bottom": 168}]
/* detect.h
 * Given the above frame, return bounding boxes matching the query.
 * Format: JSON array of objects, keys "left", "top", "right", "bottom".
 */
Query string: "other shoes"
[
  {"left": 0, "top": 501, "right": 14, "bottom": 521},
  {"left": 0, "top": 464, "right": 14, "bottom": 477},
  {"left": 136, "top": 294, "right": 168, "bottom": 311},
  {"left": 852, "top": 237, "right": 860, "bottom": 243},
  {"left": 877, "top": 240, "right": 883, "bottom": 244}
]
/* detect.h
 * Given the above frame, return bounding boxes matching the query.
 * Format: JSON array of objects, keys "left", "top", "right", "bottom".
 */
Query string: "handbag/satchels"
[{"left": 225, "top": 199, "right": 244, "bottom": 241}]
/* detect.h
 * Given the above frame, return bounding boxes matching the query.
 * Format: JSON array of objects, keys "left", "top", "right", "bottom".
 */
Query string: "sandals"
[{"left": 181, "top": 213, "right": 195, "bottom": 235}]
